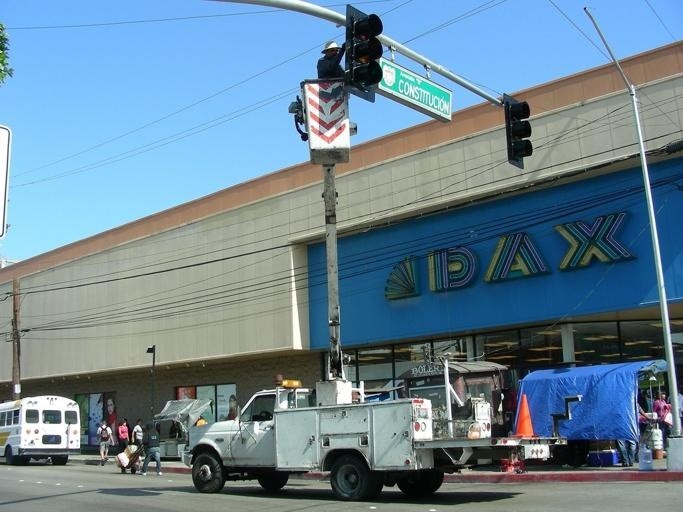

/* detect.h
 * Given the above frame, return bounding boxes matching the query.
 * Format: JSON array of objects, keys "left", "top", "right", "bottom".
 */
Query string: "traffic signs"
[{"left": 510, "top": 393, "right": 535, "bottom": 438}]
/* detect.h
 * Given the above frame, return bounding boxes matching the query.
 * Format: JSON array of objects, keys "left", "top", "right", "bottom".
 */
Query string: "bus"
[{"left": 0, "top": 395, "right": 80, "bottom": 465}]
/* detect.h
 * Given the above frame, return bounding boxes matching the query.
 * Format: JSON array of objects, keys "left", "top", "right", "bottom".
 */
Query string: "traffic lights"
[
  {"left": 344, "top": 5, "right": 383, "bottom": 104},
  {"left": 505, "top": 95, "right": 532, "bottom": 171}
]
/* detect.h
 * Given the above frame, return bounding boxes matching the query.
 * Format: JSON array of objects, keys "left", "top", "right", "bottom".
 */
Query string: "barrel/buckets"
[{"left": 638, "top": 443, "right": 654, "bottom": 470}]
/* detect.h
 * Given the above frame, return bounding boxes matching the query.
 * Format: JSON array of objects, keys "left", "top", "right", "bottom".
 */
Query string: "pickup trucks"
[{"left": 179, "top": 379, "right": 582, "bottom": 501}]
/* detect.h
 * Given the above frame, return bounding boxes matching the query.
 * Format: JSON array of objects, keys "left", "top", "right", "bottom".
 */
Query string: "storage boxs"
[{"left": 588, "top": 449, "right": 618, "bottom": 465}]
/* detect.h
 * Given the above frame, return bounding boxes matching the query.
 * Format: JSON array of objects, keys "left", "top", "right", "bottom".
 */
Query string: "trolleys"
[{"left": 120, "top": 444, "right": 144, "bottom": 474}]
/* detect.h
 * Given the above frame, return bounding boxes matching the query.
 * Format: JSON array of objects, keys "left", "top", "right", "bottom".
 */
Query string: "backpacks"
[{"left": 100, "top": 426, "right": 108, "bottom": 440}]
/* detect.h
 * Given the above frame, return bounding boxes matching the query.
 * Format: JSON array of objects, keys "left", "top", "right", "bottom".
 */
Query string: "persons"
[
  {"left": 132, "top": 418, "right": 145, "bottom": 448},
  {"left": 227, "top": 394, "right": 239, "bottom": 421},
  {"left": 142, "top": 419, "right": 161, "bottom": 475},
  {"left": 653, "top": 390, "right": 671, "bottom": 451},
  {"left": 667, "top": 388, "right": 683, "bottom": 433},
  {"left": 617, "top": 403, "right": 652, "bottom": 467},
  {"left": 95, "top": 421, "right": 115, "bottom": 465},
  {"left": 118, "top": 417, "right": 133, "bottom": 453},
  {"left": 106, "top": 397, "right": 116, "bottom": 442}
]
